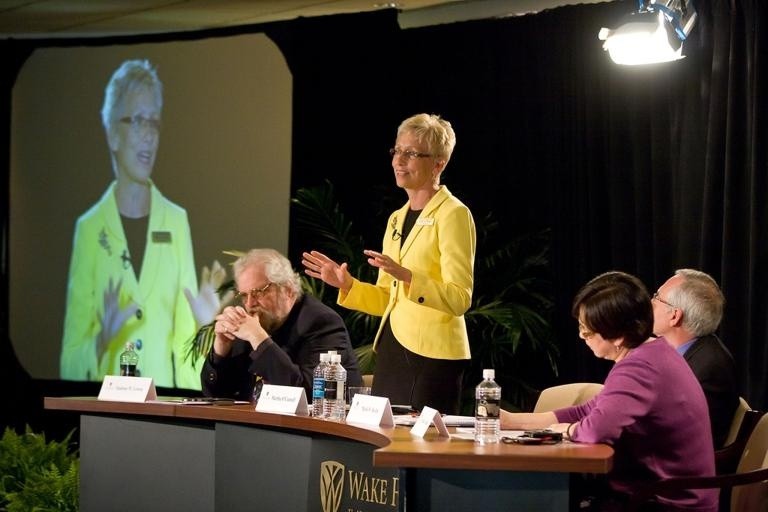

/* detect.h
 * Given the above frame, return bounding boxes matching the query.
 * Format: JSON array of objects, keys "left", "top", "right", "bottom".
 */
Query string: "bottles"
[
  {"left": 118, "top": 343, "right": 139, "bottom": 375},
  {"left": 474, "top": 369, "right": 502, "bottom": 446},
  {"left": 313, "top": 349, "right": 346, "bottom": 424}
]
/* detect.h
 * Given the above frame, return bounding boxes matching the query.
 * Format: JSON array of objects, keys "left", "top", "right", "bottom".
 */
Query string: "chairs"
[
  {"left": 531, "top": 381, "right": 604, "bottom": 415},
  {"left": 572, "top": 411, "right": 767, "bottom": 512},
  {"left": 712, "top": 397, "right": 760, "bottom": 474}
]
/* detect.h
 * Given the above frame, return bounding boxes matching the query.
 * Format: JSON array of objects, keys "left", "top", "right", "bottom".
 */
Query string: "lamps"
[{"left": 595, "top": 2, "right": 701, "bottom": 68}]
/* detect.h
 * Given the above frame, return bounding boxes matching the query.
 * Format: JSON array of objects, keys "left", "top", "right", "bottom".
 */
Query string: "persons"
[
  {"left": 199, "top": 248, "right": 364, "bottom": 404},
  {"left": 477, "top": 270, "right": 721, "bottom": 510},
  {"left": 59, "top": 59, "right": 236, "bottom": 391},
  {"left": 300, "top": 112, "right": 478, "bottom": 416},
  {"left": 650, "top": 268, "right": 741, "bottom": 452}
]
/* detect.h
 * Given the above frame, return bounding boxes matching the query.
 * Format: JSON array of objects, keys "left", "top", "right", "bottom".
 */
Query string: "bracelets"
[{"left": 566, "top": 423, "right": 575, "bottom": 442}]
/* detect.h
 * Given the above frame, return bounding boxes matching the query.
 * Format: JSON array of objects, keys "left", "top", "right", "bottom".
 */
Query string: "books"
[{"left": 393, "top": 413, "right": 478, "bottom": 427}]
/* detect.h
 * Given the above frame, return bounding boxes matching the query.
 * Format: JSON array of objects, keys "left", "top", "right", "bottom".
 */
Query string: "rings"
[{"left": 224, "top": 328, "right": 228, "bottom": 333}]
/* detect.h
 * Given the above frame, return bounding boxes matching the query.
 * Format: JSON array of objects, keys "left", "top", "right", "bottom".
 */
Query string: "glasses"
[
  {"left": 390, "top": 149, "right": 430, "bottom": 160},
  {"left": 654, "top": 293, "right": 671, "bottom": 306},
  {"left": 121, "top": 116, "right": 160, "bottom": 129},
  {"left": 235, "top": 282, "right": 271, "bottom": 304}
]
